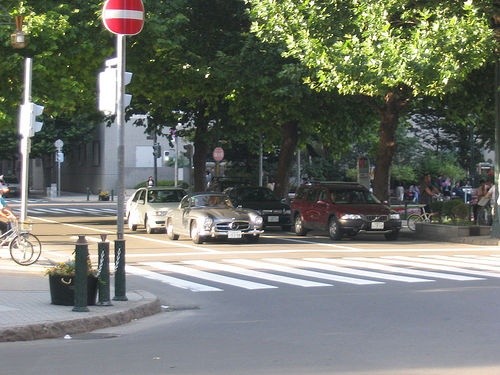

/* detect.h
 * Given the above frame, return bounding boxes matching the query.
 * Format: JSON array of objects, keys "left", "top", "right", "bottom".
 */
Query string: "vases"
[{"left": 50, "top": 275, "right": 98, "bottom": 306}]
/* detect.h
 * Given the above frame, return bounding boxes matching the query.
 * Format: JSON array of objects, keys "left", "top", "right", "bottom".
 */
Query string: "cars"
[
  {"left": 166, "top": 192, "right": 265, "bottom": 245},
  {"left": 206, "top": 177, "right": 255, "bottom": 194},
  {"left": 221, "top": 185, "right": 293, "bottom": 232},
  {"left": 124, "top": 186, "right": 187, "bottom": 234}
]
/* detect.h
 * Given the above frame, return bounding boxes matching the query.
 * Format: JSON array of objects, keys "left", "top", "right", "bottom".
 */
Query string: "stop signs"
[{"left": 101, "top": 0, "right": 146, "bottom": 36}]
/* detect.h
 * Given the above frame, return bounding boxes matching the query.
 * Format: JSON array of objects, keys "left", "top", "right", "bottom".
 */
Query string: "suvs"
[{"left": 290, "top": 179, "right": 403, "bottom": 242}]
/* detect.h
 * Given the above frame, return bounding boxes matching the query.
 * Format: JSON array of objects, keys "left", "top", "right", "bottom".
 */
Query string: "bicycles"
[
  {"left": 0, "top": 216, "right": 42, "bottom": 266},
  {"left": 406, "top": 203, "right": 455, "bottom": 231}
]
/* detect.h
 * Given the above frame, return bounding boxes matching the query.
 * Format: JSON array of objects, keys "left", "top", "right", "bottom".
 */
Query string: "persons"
[
  {"left": 396, "top": 171, "right": 495, "bottom": 227},
  {"left": 417, "top": 173, "right": 440, "bottom": 224},
  {"left": 267, "top": 178, "right": 276, "bottom": 191},
  {"left": 146, "top": 176, "right": 155, "bottom": 187},
  {"left": 0, "top": 181, "right": 15, "bottom": 237},
  {"left": 302, "top": 173, "right": 308, "bottom": 183}
]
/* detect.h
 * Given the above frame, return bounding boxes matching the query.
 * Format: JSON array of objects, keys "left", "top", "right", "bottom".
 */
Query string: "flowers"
[{"left": 43, "top": 257, "right": 106, "bottom": 287}]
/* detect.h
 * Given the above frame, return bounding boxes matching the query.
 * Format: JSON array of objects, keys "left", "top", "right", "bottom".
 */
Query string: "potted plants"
[{"left": 100, "top": 191, "right": 110, "bottom": 200}]
